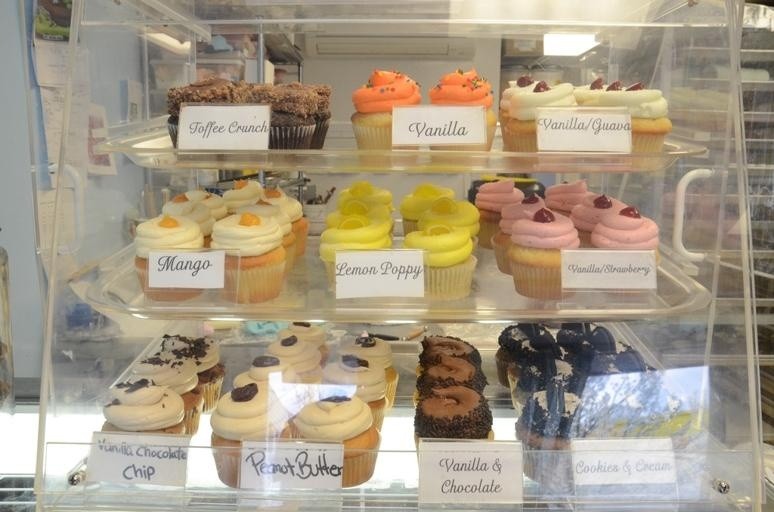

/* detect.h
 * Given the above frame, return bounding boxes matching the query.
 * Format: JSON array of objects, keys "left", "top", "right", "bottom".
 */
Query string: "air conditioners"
[
  {"left": 305, "top": 20, "right": 477, "bottom": 63},
  {"left": 140, "top": 14, "right": 304, "bottom": 225}
]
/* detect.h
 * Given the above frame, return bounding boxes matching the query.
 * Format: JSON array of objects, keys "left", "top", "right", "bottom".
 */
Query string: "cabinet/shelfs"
[
  {"left": 67, "top": 0, "right": 752, "bottom": 512},
  {"left": 677, "top": 43, "right": 773, "bottom": 449}
]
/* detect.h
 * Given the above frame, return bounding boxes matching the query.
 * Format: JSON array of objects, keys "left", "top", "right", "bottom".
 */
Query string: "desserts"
[
  {"left": 168, "top": 68, "right": 673, "bottom": 152},
  {"left": 135, "top": 179, "right": 660, "bottom": 301},
  {"left": 103, "top": 322, "right": 703, "bottom": 488}
]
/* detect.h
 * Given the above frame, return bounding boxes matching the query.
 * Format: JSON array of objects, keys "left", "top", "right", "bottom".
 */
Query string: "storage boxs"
[{"left": 503, "top": 39, "right": 544, "bottom": 65}]
